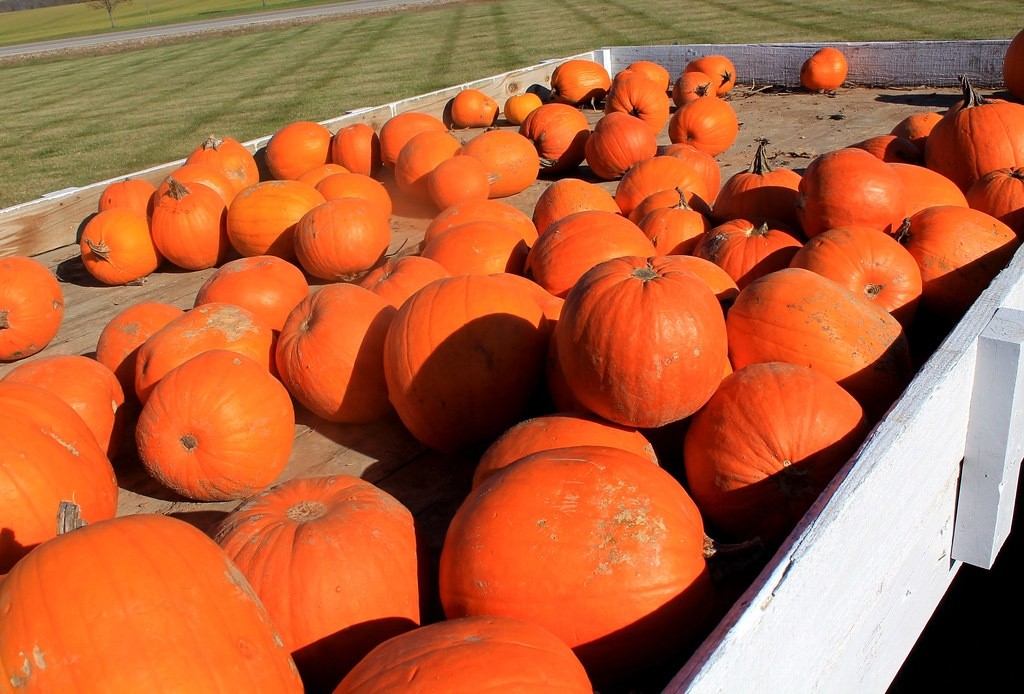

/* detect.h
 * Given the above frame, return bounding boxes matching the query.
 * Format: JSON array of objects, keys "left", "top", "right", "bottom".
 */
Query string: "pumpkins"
[{"left": 0, "top": 28, "right": 1024, "bottom": 694}]
